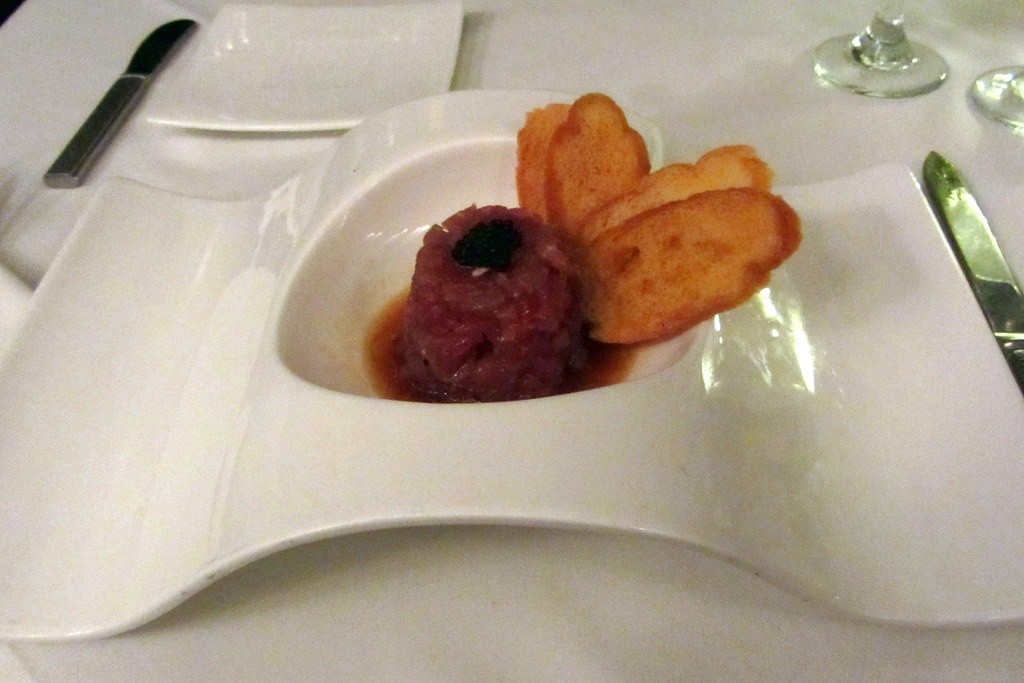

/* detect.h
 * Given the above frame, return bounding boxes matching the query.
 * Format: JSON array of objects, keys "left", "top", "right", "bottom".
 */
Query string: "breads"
[{"left": 516, "top": 94, "right": 802, "bottom": 389}]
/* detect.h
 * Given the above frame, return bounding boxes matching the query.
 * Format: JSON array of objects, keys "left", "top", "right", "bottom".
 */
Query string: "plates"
[
  {"left": 147, "top": 0, "right": 464, "bottom": 132},
  {"left": 1, "top": 91, "right": 1024, "bottom": 643}
]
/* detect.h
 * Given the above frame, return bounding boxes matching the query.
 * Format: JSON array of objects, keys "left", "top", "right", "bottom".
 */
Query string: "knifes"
[
  {"left": 923, "top": 151, "right": 1024, "bottom": 389},
  {"left": 43, "top": 19, "right": 198, "bottom": 188}
]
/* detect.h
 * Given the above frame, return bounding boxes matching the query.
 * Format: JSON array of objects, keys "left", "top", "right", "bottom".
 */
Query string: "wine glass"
[
  {"left": 971, "top": 65, "right": 1023, "bottom": 126},
  {"left": 812, "top": 1, "right": 949, "bottom": 99}
]
